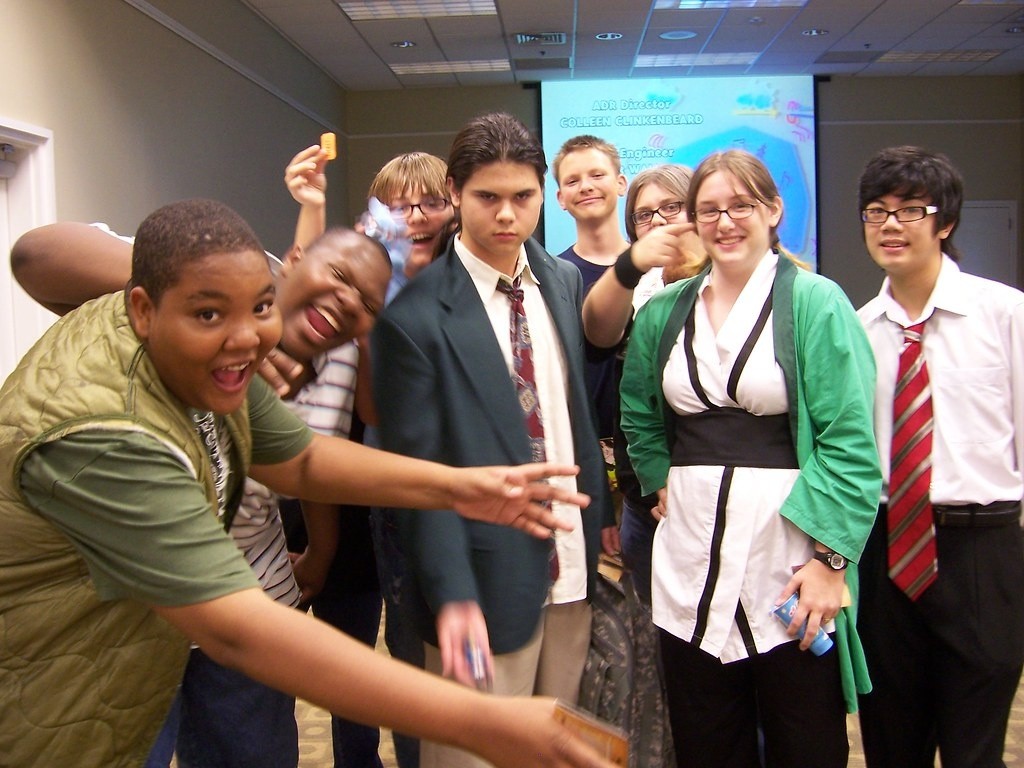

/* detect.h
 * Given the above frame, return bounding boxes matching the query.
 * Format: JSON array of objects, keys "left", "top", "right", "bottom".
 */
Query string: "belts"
[{"left": 878, "top": 505, "right": 1022, "bottom": 530}]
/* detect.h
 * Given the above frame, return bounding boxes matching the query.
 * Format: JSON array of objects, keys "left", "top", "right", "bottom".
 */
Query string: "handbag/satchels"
[{"left": 576, "top": 549, "right": 679, "bottom": 768}]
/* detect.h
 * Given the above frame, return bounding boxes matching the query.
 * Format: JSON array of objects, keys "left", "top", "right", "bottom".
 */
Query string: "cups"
[{"left": 771, "top": 591, "right": 834, "bottom": 657}]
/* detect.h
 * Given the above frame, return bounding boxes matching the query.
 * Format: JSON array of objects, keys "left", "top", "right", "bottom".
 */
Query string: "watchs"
[{"left": 812, "top": 550, "right": 848, "bottom": 570}]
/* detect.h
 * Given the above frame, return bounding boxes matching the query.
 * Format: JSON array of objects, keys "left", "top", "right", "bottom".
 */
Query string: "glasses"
[
  {"left": 630, "top": 201, "right": 685, "bottom": 226},
  {"left": 860, "top": 205, "right": 938, "bottom": 225},
  {"left": 690, "top": 201, "right": 762, "bottom": 225},
  {"left": 390, "top": 198, "right": 453, "bottom": 221}
]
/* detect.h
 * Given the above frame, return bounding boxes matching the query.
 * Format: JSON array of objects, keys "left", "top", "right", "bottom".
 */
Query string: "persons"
[
  {"left": 0, "top": 198, "right": 617, "bottom": 768},
  {"left": 370, "top": 113, "right": 621, "bottom": 768},
  {"left": 9, "top": 222, "right": 391, "bottom": 768},
  {"left": 600, "top": 440, "right": 615, "bottom": 464},
  {"left": 284, "top": 145, "right": 458, "bottom": 768},
  {"left": 619, "top": 149, "right": 882, "bottom": 768},
  {"left": 554, "top": 134, "right": 631, "bottom": 434},
  {"left": 581, "top": 166, "right": 703, "bottom": 606},
  {"left": 855, "top": 145, "right": 1024, "bottom": 768}
]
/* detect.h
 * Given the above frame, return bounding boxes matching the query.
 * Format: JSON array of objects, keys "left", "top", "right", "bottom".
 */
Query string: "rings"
[{"left": 822, "top": 617, "right": 830, "bottom": 624}]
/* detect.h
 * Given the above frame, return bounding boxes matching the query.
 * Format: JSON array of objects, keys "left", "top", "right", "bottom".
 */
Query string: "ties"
[
  {"left": 886, "top": 321, "right": 939, "bottom": 603},
  {"left": 496, "top": 273, "right": 561, "bottom": 589}
]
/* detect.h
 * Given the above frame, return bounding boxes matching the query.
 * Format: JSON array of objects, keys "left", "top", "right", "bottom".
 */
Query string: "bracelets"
[{"left": 616, "top": 247, "right": 645, "bottom": 287}]
